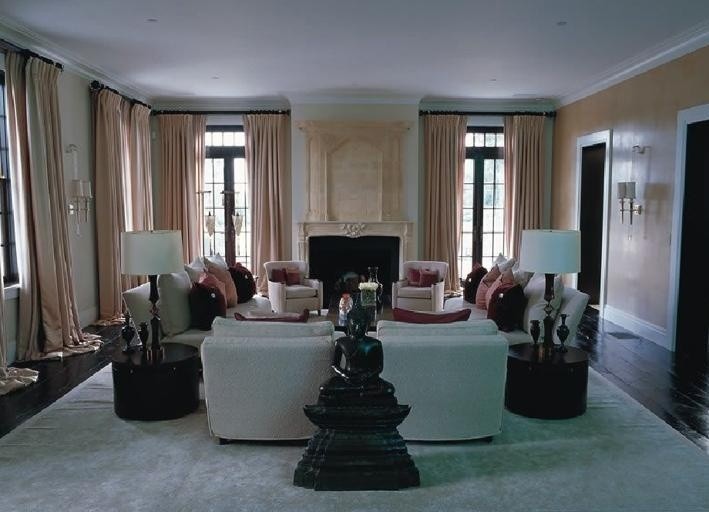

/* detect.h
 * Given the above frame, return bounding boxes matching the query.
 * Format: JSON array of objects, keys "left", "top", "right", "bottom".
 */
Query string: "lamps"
[
  {"left": 66, "top": 175, "right": 94, "bottom": 227},
  {"left": 518, "top": 228, "right": 583, "bottom": 351},
  {"left": 120, "top": 224, "right": 186, "bottom": 355},
  {"left": 196, "top": 189, "right": 216, "bottom": 236},
  {"left": 617, "top": 181, "right": 644, "bottom": 226},
  {"left": 220, "top": 190, "right": 244, "bottom": 236}
]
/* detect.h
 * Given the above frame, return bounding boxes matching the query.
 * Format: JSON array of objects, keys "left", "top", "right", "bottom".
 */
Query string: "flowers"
[{"left": 358, "top": 282, "right": 379, "bottom": 306}]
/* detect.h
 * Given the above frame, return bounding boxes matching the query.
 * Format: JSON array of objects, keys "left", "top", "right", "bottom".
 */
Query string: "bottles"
[
  {"left": 338, "top": 267, "right": 383, "bottom": 326},
  {"left": 530, "top": 320, "right": 541, "bottom": 346},
  {"left": 138, "top": 320, "right": 149, "bottom": 351},
  {"left": 557, "top": 314, "right": 568, "bottom": 352},
  {"left": 122, "top": 311, "right": 136, "bottom": 353}
]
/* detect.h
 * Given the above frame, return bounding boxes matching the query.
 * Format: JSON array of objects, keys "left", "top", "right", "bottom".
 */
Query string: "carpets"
[{"left": 1, "top": 345, "right": 709, "bottom": 512}]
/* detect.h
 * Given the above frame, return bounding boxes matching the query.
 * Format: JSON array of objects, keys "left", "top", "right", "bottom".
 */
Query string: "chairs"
[
  {"left": 363, "top": 318, "right": 510, "bottom": 444},
  {"left": 391, "top": 259, "right": 449, "bottom": 312},
  {"left": 262, "top": 259, "right": 324, "bottom": 317},
  {"left": 199, "top": 314, "right": 339, "bottom": 448}
]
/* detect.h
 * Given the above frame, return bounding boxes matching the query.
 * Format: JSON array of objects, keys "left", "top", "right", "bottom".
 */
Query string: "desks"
[
  {"left": 505, "top": 340, "right": 591, "bottom": 421},
  {"left": 110, "top": 344, "right": 202, "bottom": 423}
]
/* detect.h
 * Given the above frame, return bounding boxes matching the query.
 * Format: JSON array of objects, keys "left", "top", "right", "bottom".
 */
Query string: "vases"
[
  {"left": 338, "top": 292, "right": 353, "bottom": 313},
  {"left": 366, "top": 266, "right": 384, "bottom": 296},
  {"left": 361, "top": 290, "right": 378, "bottom": 308}
]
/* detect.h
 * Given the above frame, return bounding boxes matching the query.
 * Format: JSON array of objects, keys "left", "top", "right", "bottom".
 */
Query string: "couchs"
[
  {"left": 445, "top": 276, "right": 590, "bottom": 348},
  {"left": 121, "top": 263, "right": 271, "bottom": 372}
]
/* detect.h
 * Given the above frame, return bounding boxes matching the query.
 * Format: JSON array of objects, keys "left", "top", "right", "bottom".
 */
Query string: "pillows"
[
  {"left": 156, "top": 252, "right": 256, "bottom": 338},
  {"left": 392, "top": 307, "right": 472, "bottom": 325},
  {"left": 233, "top": 307, "right": 311, "bottom": 325},
  {"left": 462, "top": 253, "right": 564, "bottom": 338},
  {"left": 419, "top": 269, "right": 439, "bottom": 287},
  {"left": 406, "top": 266, "right": 420, "bottom": 287},
  {"left": 285, "top": 268, "right": 301, "bottom": 286},
  {"left": 272, "top": 268, "right": 287, "bottom": 283}
]
